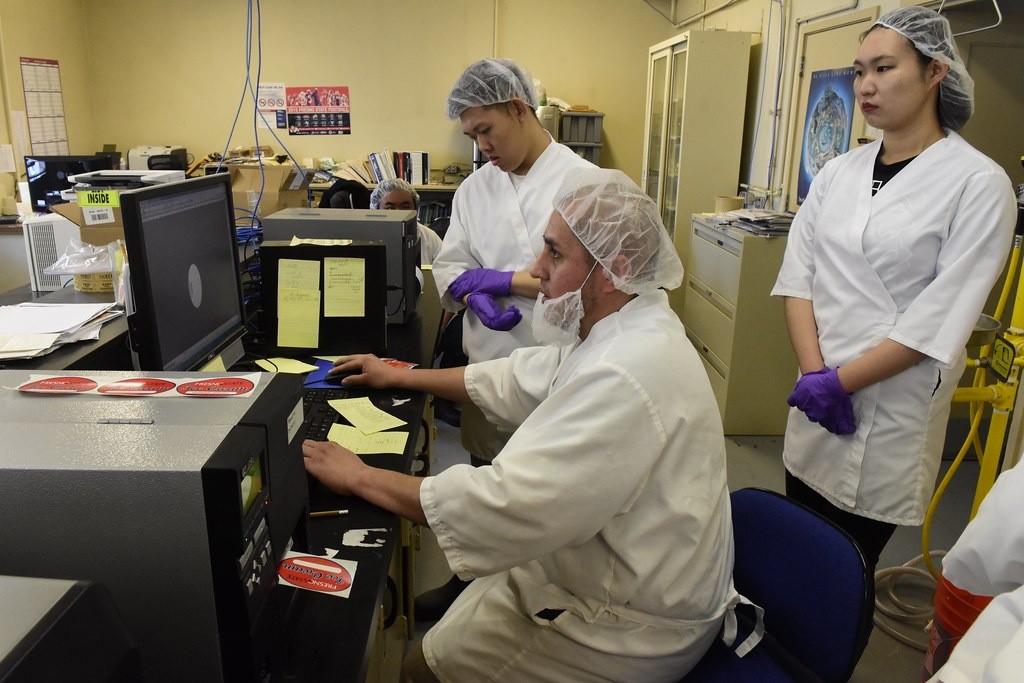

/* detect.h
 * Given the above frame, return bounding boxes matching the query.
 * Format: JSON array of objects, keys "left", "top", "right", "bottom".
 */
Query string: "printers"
[{"left": 61, "top": 169, "right": 186, "bottom": 204}]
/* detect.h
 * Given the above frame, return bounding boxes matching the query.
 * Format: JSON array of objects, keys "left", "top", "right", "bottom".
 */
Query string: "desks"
[
  {"left": 0, "top": 220, "right": 432, "bottom": 683},
  {"left": 308, "top": 171, "right": 466, "bottom": 192}
]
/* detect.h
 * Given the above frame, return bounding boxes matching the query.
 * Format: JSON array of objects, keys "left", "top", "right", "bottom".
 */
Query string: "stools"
[{"left": 687, "top": 485, "right": 872, "bottom": 683}]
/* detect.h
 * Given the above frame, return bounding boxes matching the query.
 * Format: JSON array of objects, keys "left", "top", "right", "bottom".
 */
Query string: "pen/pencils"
[{"left": 309, "top": 510, "right": 349, "bottom": 517}]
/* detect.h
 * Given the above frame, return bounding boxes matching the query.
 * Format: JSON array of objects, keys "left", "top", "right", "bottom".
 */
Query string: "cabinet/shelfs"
[
  {"left": 355, "top": 308, "right": 449, "bottom": 682},
  {"left": 641, "top": 30, "right": 799, "bottom": 436}
]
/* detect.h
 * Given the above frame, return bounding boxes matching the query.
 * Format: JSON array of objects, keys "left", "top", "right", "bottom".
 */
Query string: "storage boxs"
[
  {"left": 50, "top": 162, "right": 317, "bottom": 243},
  {"left": 559, "top": 111, "right": 605, "bottom": 167}
]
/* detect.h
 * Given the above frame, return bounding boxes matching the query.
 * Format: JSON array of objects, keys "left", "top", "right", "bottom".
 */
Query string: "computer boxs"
[{"left": 258, "top": 240, "right": 388, "bottom": 353}]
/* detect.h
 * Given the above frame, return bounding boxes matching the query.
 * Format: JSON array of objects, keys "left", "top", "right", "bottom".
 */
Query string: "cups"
[
  {"left": 114, "top": 249, "right": 128, "bottom": 281},
  {"left": 715, "top": 196, "right": 746, "bottom": 213}
]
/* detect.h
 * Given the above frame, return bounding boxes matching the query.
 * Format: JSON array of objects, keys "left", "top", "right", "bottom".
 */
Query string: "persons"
[
  {"left": 412, "top": 59, "right": 600, "bottom": 622},
  {"left": 371, "top": 178, "right": 442, "bottom": 266},
  {"left": 301, "top": 167, "right": 766, "bottom": 683},
  {"left": 771, "top": 7, "right": 1018, "bottom": 571},
  {"left": 925, "top": 453, "right": 1024, "bottom": 683}
]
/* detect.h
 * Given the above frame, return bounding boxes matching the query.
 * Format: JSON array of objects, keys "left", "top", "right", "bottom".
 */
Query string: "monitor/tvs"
[
  {"left": 23, "top": 155, "right": 112, "bottom": 215},
  {"left": 119, "top": 171, "right": 249, "bottom": 374}
]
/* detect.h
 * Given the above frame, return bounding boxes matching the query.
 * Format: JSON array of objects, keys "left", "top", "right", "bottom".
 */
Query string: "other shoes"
[{"left": 415, "top": 585, "right": 450, "bottom": 616}]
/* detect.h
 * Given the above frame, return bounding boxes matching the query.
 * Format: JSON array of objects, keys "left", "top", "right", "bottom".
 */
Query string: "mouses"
[{"left": 326, "top": 369, "right": 364, "bottom": 385}]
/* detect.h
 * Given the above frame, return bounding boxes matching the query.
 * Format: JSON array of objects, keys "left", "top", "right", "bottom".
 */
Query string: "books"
[{"left": 336, "top": 149, "right": 429, "bottom": 185}]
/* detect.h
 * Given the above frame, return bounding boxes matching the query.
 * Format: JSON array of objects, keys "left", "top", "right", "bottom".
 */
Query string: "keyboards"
[{"left": 301, "top": 384, "right": 354, "bottom": 445}]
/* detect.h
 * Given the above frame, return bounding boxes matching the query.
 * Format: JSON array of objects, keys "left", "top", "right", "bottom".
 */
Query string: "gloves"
[
  {"left": 466, "top": 292, "right": 523, "bottom": 331},
  {"left": 788, "top": 366, "right": 854, "bottom": 423},
  {"left": 802, "top": 367, "right": 856, "bottom": 435},
  {"left": 449, "top": 268, "right": 514, "bottom": 300}
]
